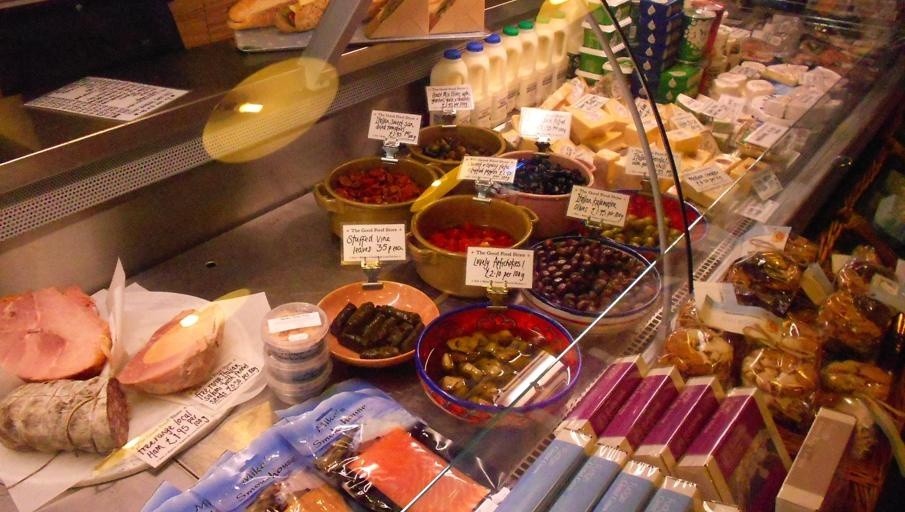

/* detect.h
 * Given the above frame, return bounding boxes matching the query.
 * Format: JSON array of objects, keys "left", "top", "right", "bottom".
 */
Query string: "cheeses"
[{"left": 502, "top": 62, "right": 850, "bottom": 228}]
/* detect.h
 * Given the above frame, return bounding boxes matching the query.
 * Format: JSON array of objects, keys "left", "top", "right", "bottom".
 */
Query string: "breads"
[{"left": 669, "top": 252, "right": 893, "bottom": 418}]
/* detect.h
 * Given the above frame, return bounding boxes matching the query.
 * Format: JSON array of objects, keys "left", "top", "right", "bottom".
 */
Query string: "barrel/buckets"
[{"left": 430, "top": 0, "right": 581, "bottom": 129}]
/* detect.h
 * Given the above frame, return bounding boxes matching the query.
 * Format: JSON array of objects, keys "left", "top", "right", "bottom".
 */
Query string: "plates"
[{"left": 316, "top": 281, "right": 440, "bottom": 369}]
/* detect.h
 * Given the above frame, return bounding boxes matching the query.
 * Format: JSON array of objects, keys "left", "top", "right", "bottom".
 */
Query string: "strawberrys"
[{"left": 430, "top": 222, "right": 514, "bottom": 252}]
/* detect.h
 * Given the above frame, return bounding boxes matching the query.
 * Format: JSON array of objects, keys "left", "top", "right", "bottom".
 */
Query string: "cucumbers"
[{"left": 330, "top": 301, "right": 425, "bottom": 359}]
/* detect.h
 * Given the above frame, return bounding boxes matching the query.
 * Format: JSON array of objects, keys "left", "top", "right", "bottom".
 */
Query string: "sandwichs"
[{"left": 364, "top": 0, "right": 486, "bottom": 37}]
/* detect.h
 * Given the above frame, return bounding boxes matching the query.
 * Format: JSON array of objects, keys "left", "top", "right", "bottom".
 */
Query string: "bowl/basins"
[
  {"left": 311, "top": 153, "right": 444, "bottom": 241},
  {"left": 515, "top": 237, "right": 663, "bottom": 338},
  {"left": 401, "top": 195, "right": 538, "bottom": 302},
  {"left": 405, "top": 121, "right": 510, "bottom": 168},
  {"left": 416, "top": 300, "right": 586, "bottom": 428},
  {"left": 493, "top": 148, "right": 600, "bottom": 237},
  {"left": 584, "top": 187, "right": 707, "bottom": 263}
]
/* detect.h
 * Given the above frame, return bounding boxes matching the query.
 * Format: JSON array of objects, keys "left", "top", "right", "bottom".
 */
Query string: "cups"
[{"left": 678, "top": 2, "right": 725, "bottom": 63}]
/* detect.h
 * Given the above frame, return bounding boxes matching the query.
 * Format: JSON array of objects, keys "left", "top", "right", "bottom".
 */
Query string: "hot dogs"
[{"left": 226, "top": 2, "right": 327, "bottom": 32}]
